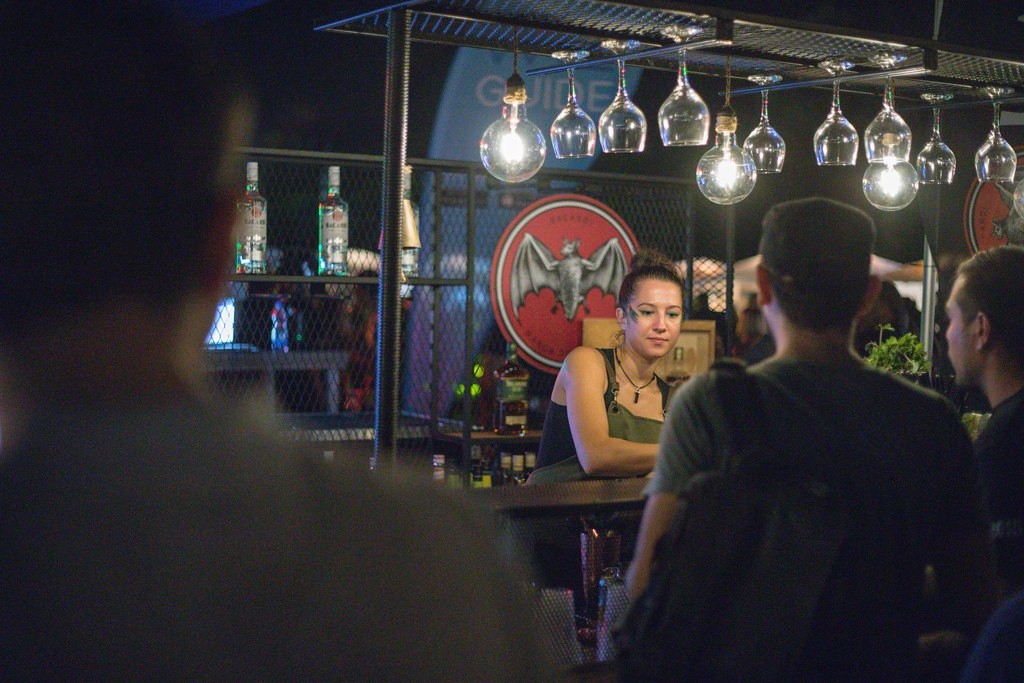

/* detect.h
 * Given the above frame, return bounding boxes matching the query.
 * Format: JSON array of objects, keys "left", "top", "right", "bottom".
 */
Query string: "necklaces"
[{"left": 615, "top": 346, "right": 656, "bottom": 403}]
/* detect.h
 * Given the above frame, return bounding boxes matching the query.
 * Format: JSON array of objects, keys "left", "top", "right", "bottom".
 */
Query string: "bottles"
[
  {"left": 666, "top": 346, "right": 694, "bottom": 388},
  {"left": 317, "top": 166, "right": 350, "bottom": 277},
  {"left": 490, "top": 341, "right": 530, "bottom": 436},
  {"left": 434, "top": 446, "right": 537, "bottom": 490},
  {"left": 236, "top": 161, "right": 268, "bottom": 275},
  {"left": 269, "top": 301, "right": 291, "bottom": 353}
]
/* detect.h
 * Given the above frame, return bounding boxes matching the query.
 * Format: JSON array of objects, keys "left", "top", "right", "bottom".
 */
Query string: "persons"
[
  {"left": 533, "top": 250, "right": 686, "bottom": 666},
  {"left": 0, "top": 0, "right": 545, "bottom": 683},
  {"left": 623, "top": 197, "right": 1024, "bottom": 683}
]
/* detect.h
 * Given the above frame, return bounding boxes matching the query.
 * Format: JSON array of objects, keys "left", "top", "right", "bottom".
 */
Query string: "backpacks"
[{"left": 607, "top": 357, "right": 851, "bottom": 683}]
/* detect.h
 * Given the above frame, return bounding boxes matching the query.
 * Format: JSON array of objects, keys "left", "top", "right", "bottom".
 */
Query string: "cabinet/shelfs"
[{"left": 226, "top": 146, "right": 735, "bottom": 490}]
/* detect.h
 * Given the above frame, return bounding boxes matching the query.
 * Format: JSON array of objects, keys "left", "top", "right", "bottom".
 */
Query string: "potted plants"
[{"left": 863, "top": 322, "right": 937, "bottom": 385}]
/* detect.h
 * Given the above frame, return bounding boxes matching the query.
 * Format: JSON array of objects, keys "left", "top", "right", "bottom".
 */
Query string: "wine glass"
[
  {"left": 863, "top": 51, "right": 911, "bottom": 164},
  {"left": 656, "top": 23, "right": 711, "bottom": 147},
  {"left": 550, "top": 49, "right": 596, "bottom": 160},
  {"left": 916, "top": 91, "right": 957, "bottom": 185},
  {"left": 813, "top": 59, "right": 859, "bottom": 167},
  {"left": 743, "top": 74, "right": 785, "bottom": 176},
  {"left": 975, "top": 84, "right": 1017, "bottom": 183},
  {"left": 598, "top": 38, "right": 647, "bottom": 154}
]
